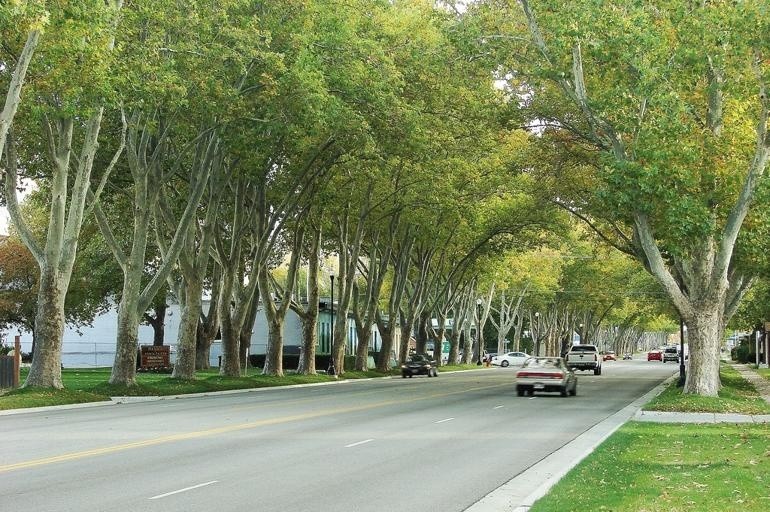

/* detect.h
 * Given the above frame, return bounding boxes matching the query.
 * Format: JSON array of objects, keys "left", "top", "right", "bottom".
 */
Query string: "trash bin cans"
[
  {"left": 170, "top": 346, "right": 177, "bottom": 365},
  {"left": 1, "top": 355, "right": 15, "bottom": 389}
]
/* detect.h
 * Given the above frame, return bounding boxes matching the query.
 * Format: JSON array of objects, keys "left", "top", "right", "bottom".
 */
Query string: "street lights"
[
  {"left": 326, "top": 266, "right": 337, "bottom": 375},
  {"left": 535, "top": 311, "right": 540, "bottom": 357},
  {"left": 477, "top": 298, "right": 482, "bottom": 365},
  {"left": 580, "top": 324, "right": 583, "bottom": 343},
  {"left": 503, "top": 338, "right": 510, "bottom": 353}
]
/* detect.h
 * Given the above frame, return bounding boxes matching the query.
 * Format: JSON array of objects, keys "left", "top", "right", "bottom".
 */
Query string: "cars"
[
  {"left": 411, "top": 343, "right": 434, "bottom": 358},
  {"left": 459, "top": 344, "right": 601, "bottom": 398},
  {"left": 647, "top": 344, "right": 688, "bottom": 363},
  {"left": 400, "top": 353, "right": 438, "bottom": 377},
  {"left": 623, "top": 353, "right": 632, "bottom": 360},
  {"left": 603, "top": 350, "right": 617, "bottom": 361}
]
